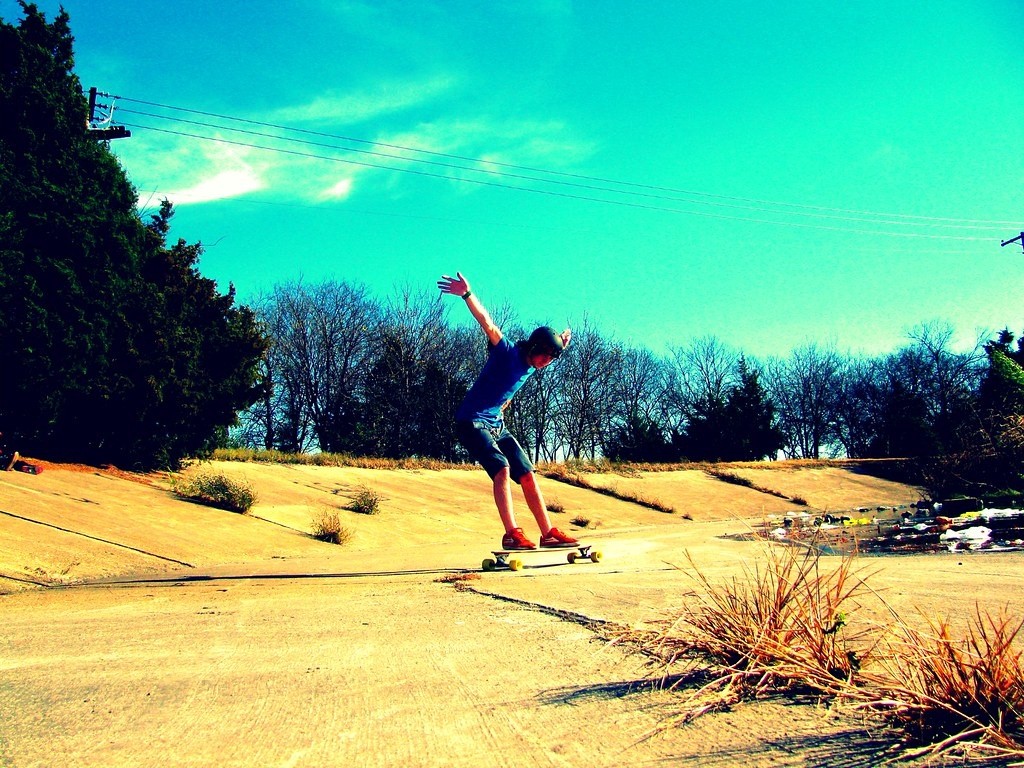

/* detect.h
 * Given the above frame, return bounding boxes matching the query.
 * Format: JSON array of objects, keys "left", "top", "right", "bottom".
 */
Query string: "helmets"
[{"left": 529, "top": 326, "right": 563, "bottom": 360}]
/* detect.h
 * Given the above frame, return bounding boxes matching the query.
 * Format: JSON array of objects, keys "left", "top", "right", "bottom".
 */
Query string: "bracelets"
[{"left": 460, "top": 290, "right": 472, "bottom": 300}]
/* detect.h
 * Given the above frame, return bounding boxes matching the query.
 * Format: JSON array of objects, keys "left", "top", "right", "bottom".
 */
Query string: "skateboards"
[{"left": 481, "top": 543, "right": 604, "bottom": 570}]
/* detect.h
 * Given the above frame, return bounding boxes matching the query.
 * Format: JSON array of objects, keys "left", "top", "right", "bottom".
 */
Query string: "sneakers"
[
  {"left": 540, "top": 526, "right": 580, "bottom": 548},
  {"left": 502, "top": 527, "right": 537, "bottom": 550}
]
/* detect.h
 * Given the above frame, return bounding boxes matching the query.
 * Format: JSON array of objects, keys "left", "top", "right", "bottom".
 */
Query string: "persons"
[{"left": 440, "top": 270, "right": 583, "bottom": 550}]
